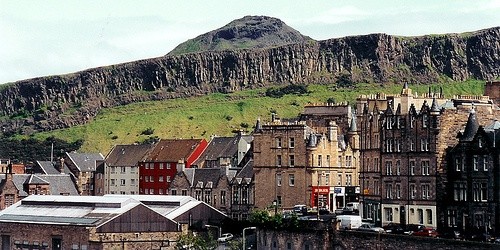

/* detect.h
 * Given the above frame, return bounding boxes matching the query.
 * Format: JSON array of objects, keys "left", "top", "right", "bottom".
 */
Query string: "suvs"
[{"left": 217, "top": 233, "right": 234, "bottom": 243}]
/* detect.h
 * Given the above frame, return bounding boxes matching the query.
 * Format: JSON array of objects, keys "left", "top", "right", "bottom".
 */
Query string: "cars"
[
  {"left": 356, "top": 223, "right": 386, "bottom": 233},
  {"left": 334, "top": 207, "right": 347, "bottom": 215},
  {"left": 382, "top": 222, "right": 500, "bottom": 244}
]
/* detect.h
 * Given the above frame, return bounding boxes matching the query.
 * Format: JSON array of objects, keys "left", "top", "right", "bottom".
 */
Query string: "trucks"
[
  {"left": 331, "top": 214, "right": 362, "bottom": 230},
  {"left": 345, "top": 202, "right": 359, "bottom": 214},
  {"left": 293, "top": 204, "right": 307, "bottom": 217}
]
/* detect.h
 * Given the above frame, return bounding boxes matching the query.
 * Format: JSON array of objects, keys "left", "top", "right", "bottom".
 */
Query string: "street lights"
[
  {"left": 275, "top": 170, "right": 286, "bottom": 218},
  {"left": 205, "top": 225, "right": 222, "bottom": 238},
  {"left": 243, "top": 226, "right": 257, "bottom": 250}
]
